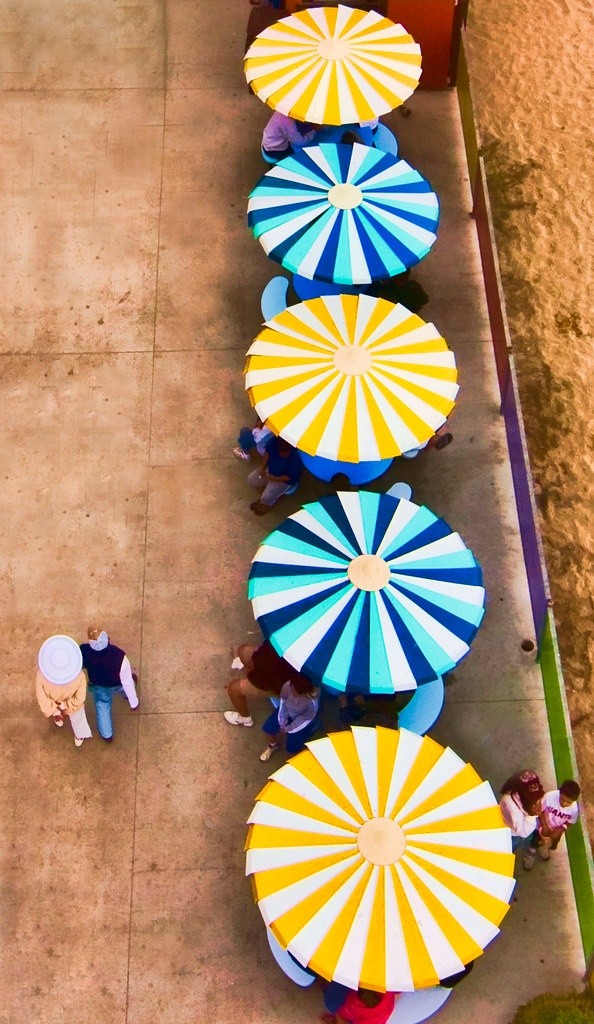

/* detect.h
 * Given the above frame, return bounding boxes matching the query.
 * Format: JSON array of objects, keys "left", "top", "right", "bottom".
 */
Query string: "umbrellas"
[
  {"left": 243, "top": 725, "right": 516, "bottom": 994},
  {"left": 242, "top": 293, "right": 460, "bottom": 464},
  {"left": 247, "top": 141, "right": 440, "bottom": 285},
  {"left": 248, "top": 491, "right": 486, "bottom": 703},
  {"left": 242, "top": 4, "right": 423, "bottom": 126}
]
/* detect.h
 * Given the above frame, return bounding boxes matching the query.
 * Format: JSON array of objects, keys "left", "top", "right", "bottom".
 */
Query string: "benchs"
[
  {"left": 382, "top": 988, "right": 451, "bottom": 1024},
  {"left": 261, "top": 276, "right": 290, "bottom": 323},
  {"left": 370, "top": 120, "right": 398, "bottom": 159},
  {"left": 265, "top": 925, "right": 315, "bottom": 987},
  {"left": 394, "top": 675, "right": 444, "bottom": 736}
]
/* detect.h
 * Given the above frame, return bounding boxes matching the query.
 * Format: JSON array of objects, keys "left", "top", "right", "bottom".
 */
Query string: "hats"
[
  {"left": 37, "top": 634, "right": 83, "bottom": 685},
  {"left": 517, "top": 769, "right": 544, "bottom": 801}
]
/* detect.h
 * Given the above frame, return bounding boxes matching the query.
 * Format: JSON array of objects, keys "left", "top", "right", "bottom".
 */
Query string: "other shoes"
[
  {"left": 53, "top": 715, "right": 63, "bottom": 727},
  {"left": 436, "top": 433, "right": 453, "bottom": 449},
  {"left": 259, "top": 747, "right": 273, "bottom": 761},
  {"left": 524, "top": 852, "right": 536, "bottom": 869},
  {"left": 73, "top": 736, "right": 84, "bottom": 747},
  {"left": 231, "top": 657, "right": 244, "bottom": 669},
  {"left": 224, "top": 711, "right": 253, "bottom": 726},
  {"left": 103, "top": 736, "right": 113, "bottom": 743},
  {"left": 538, "top": 846, "right": 550, "bottom": 860},
  {"left": 403, "top": 107, "right": 412, "bottom": 117},
  {"left": 233, "top": 447, "right": 251, "bottom": 463}
]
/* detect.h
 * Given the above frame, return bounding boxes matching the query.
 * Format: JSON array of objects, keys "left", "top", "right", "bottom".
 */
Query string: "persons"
[
  {"left": 223, "top": 640, "right": 296, "bottom": 727},
  {"left": 400, "top": 104, "right": 413, "bottom": 117},
  {"left": 338, "top": 693, "right": 368, "bottom": 723},
  {"left": 232, "top": 416, "right": 273, "bottom": 464},
  {"left": 321, "top": 987, "right": 402, "bottom": 1024},
  {"left": 79, "top": 625, "right": 139, "bottom": 743},
  {"left": 310, "top": 473, "right": 358, "bottom": 500},
  {"left": 36, "top": 634, "right": 92, "bottom": 746},
  {"left": 357, "top": 116, "right": 379, "bottom": 147},
  {"left": 500, "top": 770, "right": 545, "bottom": 852},
  {"left": 250, "top": 435, "right": 305, "bottom": 515},
  {"left": 341, "top": 130, "right": 363, "bottom": 144},
  {"left": 261, "top": 111, "right": 323, "bottom": 160},
  {"left": 400, "top": 281, "right": 430, "bottom": 314},
  {"left": 523, "top": 779, "right": 580, "bottom": 871},
  {"left": 260, "top": 672, "right": 322, "bottom": 762}
]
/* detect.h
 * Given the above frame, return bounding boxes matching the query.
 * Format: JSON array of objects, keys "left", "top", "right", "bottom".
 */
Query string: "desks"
[
  {"left": 298, "top": 452, "right": 393, "bottom": 487},
  {"left": 289, "top": 125, "right": 373, "bottom": 152}
]
